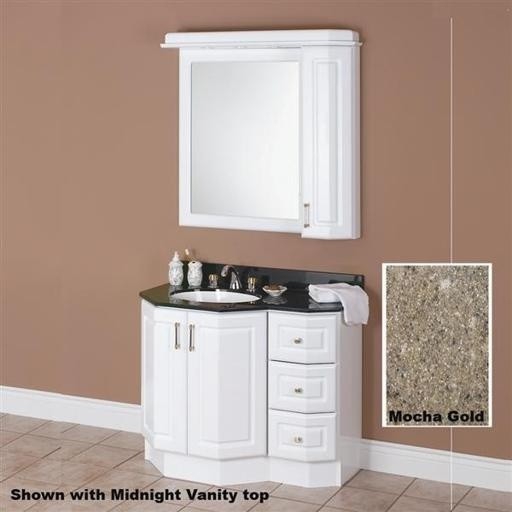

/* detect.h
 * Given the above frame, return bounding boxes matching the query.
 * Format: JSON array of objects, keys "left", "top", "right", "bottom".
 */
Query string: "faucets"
[{"left": 219, "top": 264, "right": 244, "bottom": 291}]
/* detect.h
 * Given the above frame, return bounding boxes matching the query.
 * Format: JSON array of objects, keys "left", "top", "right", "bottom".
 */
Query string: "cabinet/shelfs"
[
  {"left": 267, "top": 307, "right": 364, "bottom": 490},
  {"left": 139, "top": 297, "right": 269, "bottom": 486},
  {"left": 300, "top": 40, "right": 364, "bottom": 242}
]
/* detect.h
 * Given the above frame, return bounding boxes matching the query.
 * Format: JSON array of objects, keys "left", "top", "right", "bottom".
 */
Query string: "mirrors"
[{"left": 176, "top": 47, "right": 302, "bottom": 237}]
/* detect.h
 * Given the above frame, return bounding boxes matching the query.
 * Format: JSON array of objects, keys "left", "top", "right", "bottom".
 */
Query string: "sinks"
[{"left": 168, "top": 287, "right": 262, "bottom": 304}]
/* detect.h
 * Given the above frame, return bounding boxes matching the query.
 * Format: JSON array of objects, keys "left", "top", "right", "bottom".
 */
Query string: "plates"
[{"left": 263, "top": 285, "right": 287, "bottom": 297}]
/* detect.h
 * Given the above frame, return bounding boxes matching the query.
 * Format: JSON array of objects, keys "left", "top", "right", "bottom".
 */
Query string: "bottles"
[
  {"left": 168, "top": 252, "right": 184, "bottom": 285},
  {"left": 187, "top": 261, "right": 203, "bottom": 286}
]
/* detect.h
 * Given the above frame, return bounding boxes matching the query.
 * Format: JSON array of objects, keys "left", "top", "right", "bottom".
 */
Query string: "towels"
[{"left": 307, "top": 280, "right": 370, "bottom": 328}]
